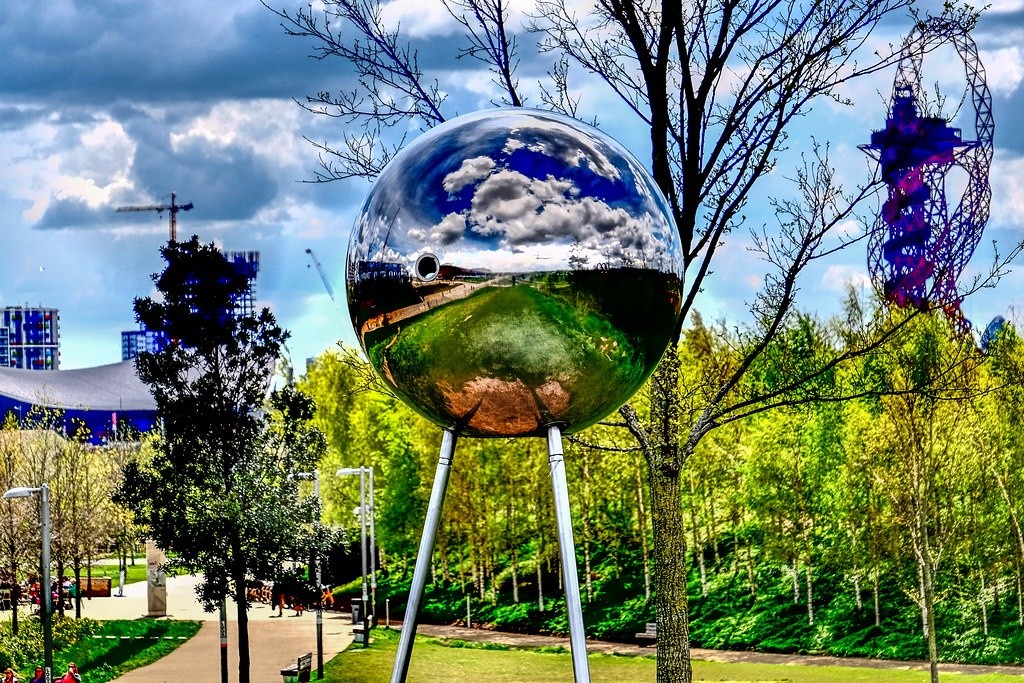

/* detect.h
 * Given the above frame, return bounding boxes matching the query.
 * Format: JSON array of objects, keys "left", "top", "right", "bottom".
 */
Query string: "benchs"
[
  {"left": 635, "top": 623, "right": 657, "bottom": 647},
  {"left": 353, "top": 615, "right": 374, "bottom": 643},
  {"left": 280, "top": 652, "right": 312, "bottom": 683}
]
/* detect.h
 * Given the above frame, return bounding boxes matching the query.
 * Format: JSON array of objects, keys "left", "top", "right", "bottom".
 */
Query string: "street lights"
[
  {"left": 3, "top": 482, "right": 53, "bottom": 683},
  {"left": 287, "top": 470, "right": 323, "bottom": 679},
  {"left": 336, "top": 466, "right": 376, "bottom": 649}
]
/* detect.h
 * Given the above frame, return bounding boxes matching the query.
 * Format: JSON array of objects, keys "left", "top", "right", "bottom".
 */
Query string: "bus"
[{"left": 353, "top": 261, "right": 409, "bottom": 284}]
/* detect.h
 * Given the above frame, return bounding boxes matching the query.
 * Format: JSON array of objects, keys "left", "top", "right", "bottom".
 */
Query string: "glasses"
[
  {"left": 5, "top": 671, "right": 10, "bottom": 673},
  {"left": 36, "top": 670, "right": 41, "bottom": 672},
  {"left": 68, "top": 665, "right": 75, "bottom": 668}
]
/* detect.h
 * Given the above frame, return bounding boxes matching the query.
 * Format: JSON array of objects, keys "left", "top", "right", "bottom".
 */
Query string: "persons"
[
  {"left": 0, "top": 575, "right": 22, "bottom": 610},
  {"left": 0, "top": 667, "right": 19, "bottom": 683},
  {"left": 28, "top": 573, "right": 82, "bottom": 614},
  {"left": 53, "top": 662, "right": 81, "bottom": 683},
  {"left": 29, "top": 667, "right": 46, "bottom": 683}
]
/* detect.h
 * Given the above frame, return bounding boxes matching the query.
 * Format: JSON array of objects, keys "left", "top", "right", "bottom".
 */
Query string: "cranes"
[
  {"left": 304, "top": 248, "right": 339, "bottom": 302},
  {"left": 115, "top": 191, "right": 193, "bottom": 241}
]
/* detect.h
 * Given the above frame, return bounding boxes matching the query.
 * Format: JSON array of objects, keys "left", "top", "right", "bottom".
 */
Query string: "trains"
[{"left": 436, "top": 265, "right": 473, "bottom": 279}]
